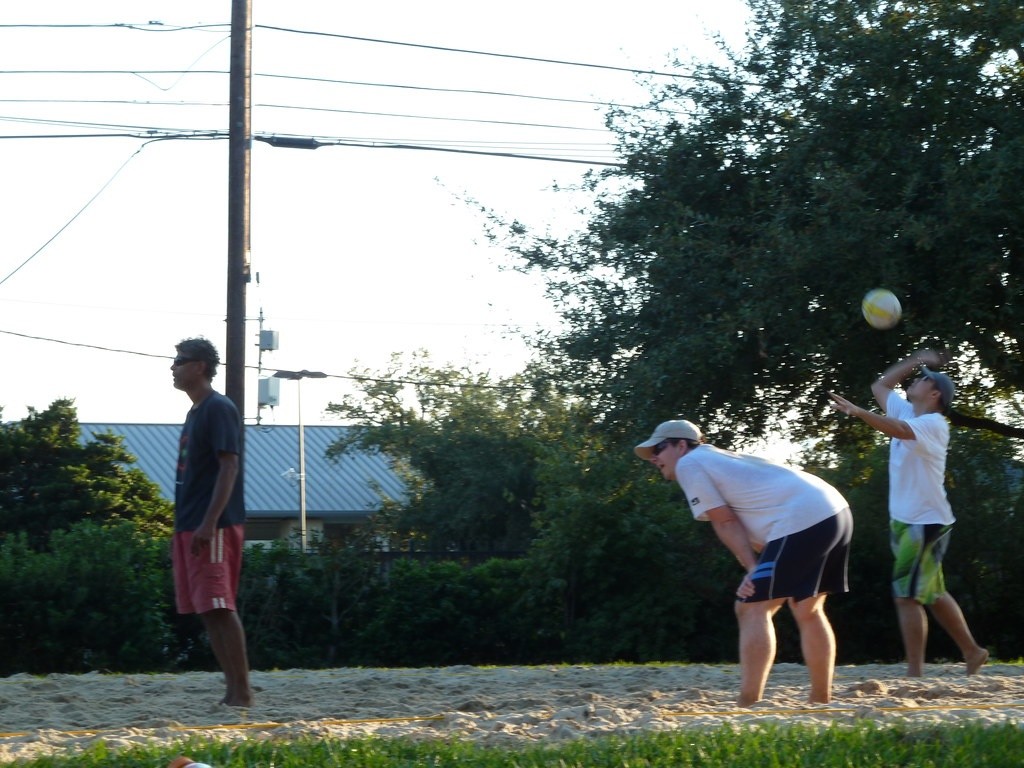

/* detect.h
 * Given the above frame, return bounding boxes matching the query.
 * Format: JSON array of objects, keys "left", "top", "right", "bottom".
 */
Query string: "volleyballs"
[{"left": 862, "top": 287, "right": 902, "bottom": 330}]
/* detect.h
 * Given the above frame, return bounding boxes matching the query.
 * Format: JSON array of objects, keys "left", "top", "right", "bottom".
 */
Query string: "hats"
[
  {"left": 922, "top": 366, "right": 954, "bottom": 406},
  {"left": 634, "top": 420, "right": 702, "bottom": 460}
]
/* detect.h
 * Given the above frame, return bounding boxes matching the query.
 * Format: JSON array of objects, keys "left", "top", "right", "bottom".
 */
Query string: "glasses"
[
  {"left": 174, "top": 356, "right": 203, "bottom": 366},
  {"left": 655, "top": 441, "right": 677, "bottom": 455},
  {"left": 921, "top": 375, "right": 930, "bottom": 382}
]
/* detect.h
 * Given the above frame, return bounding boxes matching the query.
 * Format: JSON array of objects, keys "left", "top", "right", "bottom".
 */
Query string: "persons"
[
  {"left": 825, "top": 347, "right": 992, "bottom": 679},
  {"left": 635, "top": 416, "right": 855, "bottom": 709},
  {"left": 170, "top": 336, "right": 253, "bottom": 708}
]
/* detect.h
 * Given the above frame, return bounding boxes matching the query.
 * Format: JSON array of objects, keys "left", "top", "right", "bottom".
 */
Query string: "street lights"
[{"left": 272, "top": 370, "right": 327, "bottom": 552}]
